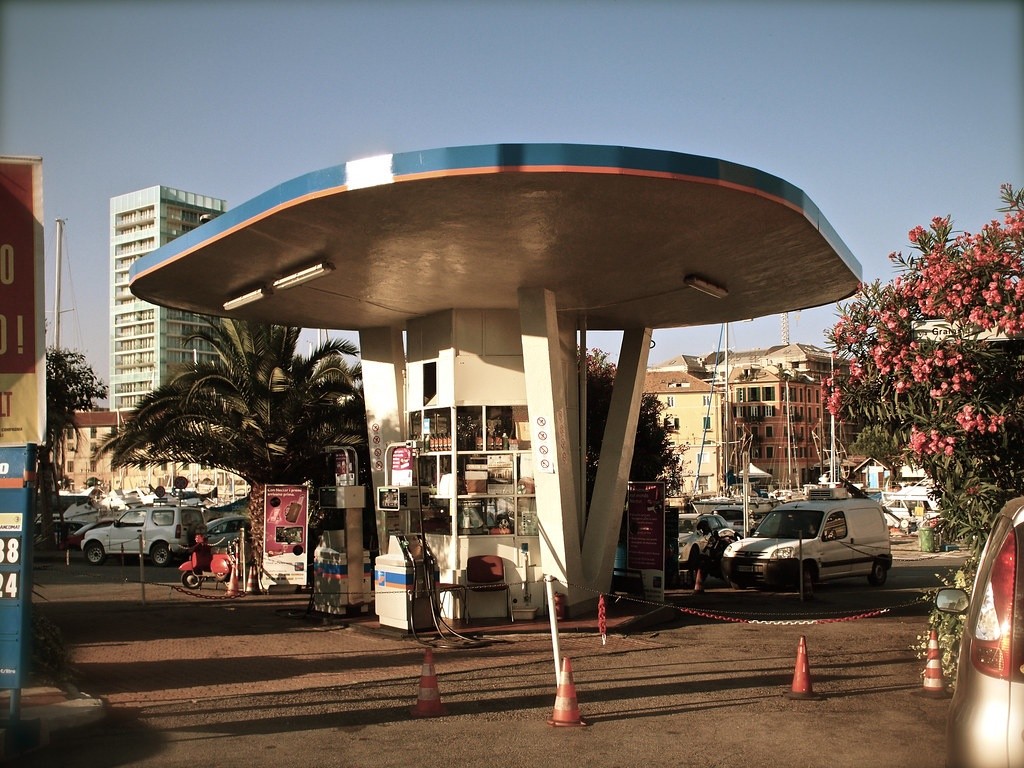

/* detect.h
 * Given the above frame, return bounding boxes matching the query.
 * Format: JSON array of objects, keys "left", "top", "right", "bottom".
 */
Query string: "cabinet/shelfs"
[
  {"left": 454, "top": 404, "right": 547, "bottom": 621},
  {"left": 401, "top": 406, "right": 455, "bottom": 623}
]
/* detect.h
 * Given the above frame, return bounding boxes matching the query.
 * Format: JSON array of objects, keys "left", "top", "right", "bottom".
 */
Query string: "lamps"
[
  {"left": 223, "top": 287, "right": 265, "bottom": 311},
  {"left": 272, "top": 261, "right": 336, "bottom": 290},
  {"left": 683, "top": 274, "right": 728, "bottom": 300}
]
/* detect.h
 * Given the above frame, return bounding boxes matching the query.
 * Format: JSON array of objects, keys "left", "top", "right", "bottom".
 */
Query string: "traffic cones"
[
  {"left": 407, "top": 646, "right": 452, "bottom": 719},
  {"left": 224, "top": 566, "right": 239, "bottom": 597},
  {"left": 911, "top": 626, "right": 955, "bottom": 699},
  {"left": 692, "top": 569, "right": 706, "bottom": 597},
  {"left": 546, "top": 656, "right": 589, "bottom": 729},
  {"left": 783, "top": 635, "right": 825, "bottom": 700},
  {"left": 246, "top": 565, "right": 259, "bottom": 592}
]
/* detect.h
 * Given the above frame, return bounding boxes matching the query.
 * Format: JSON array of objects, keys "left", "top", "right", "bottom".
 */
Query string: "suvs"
[
  {"left": 80, "top": 506, "right": 207, "bottom": 568},
  {"left": 678, "top": 512, "right": 722, "bottom": 570},
  {"left": 710, "top": 505, "right": 762, "bottom": 539},
  {"left": 720, "top": 488, "right": 893, "bottom": 597}
]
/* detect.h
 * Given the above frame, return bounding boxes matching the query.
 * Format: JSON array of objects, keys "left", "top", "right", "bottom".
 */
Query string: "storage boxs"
[{"left": 512, "top": 606, "right": 538, "bottom": 620}]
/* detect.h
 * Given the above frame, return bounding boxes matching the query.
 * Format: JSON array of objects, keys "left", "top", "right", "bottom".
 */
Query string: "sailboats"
[
  {"left": 36, "top": 400, "right": 248, "bottom": 523},
  {"left": 667, "top": 323, "right": 941, "bottom": 533}
]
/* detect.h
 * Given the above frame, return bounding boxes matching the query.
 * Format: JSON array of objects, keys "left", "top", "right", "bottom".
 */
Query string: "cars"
[
  {"left": 67, "top": 518, "right": 117, "bottom": 552},
  {"left": 935, "top": 496, "right": 1024, "bottom": 768},
  {"left": 206, "top": 515, "right": 252, "bottom": 547}
]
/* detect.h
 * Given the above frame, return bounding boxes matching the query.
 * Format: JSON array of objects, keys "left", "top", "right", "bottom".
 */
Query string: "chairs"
[{"left": 465, "top": 554, "right": 514, "bottom": 624}]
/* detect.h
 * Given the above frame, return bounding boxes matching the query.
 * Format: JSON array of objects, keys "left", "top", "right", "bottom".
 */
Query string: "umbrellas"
[
  {"left": 813, "top": 456, "right": 857, "bottom": 467},
  {"left": 733, "top": 462, "right": 772, "bottom": 479}
]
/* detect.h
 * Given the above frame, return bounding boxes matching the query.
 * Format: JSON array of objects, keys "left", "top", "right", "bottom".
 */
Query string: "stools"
[{"left": 440, "top": 583, "right": 474, "bottom": 623}]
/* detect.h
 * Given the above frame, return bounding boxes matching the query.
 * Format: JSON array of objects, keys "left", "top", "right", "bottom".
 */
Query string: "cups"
[{"left": 285, "top": 503, "right": 301, "bottom": 524}]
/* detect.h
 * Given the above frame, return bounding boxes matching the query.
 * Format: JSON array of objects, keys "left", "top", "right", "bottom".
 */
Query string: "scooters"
[{"left": 180, "top": 535, "right": 232, "bottom": 589}]
